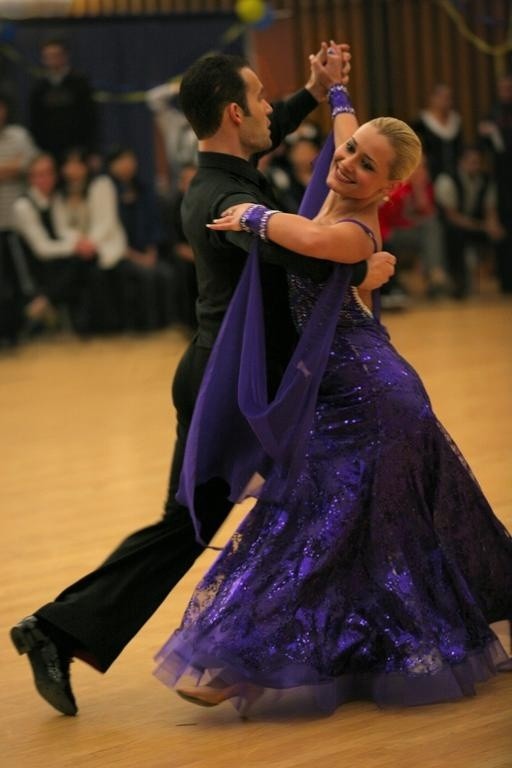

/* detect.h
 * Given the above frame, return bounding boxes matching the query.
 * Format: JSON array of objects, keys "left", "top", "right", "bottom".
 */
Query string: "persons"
[
  {"left": 259, "top": 82, "right": 512, "bottom": 313},
  {"left": 1, "top": 41, "right": 198, "bottom": 349},
  {"left": 154, "top": 40, "right": 512, "bottom": 721},
  {"left": 9, "top": 44, "right": 397, "bottom": 716}
]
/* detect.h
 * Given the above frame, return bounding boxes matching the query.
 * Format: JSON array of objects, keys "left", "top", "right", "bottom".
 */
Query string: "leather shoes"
[{"left": 9, "top": 614, "right": 78, "bottom": 718}]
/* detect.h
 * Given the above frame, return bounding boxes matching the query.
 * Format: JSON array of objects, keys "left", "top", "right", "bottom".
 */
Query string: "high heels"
[{"left": 176, "top": 673, "right": 265, "bottom": 719}]
